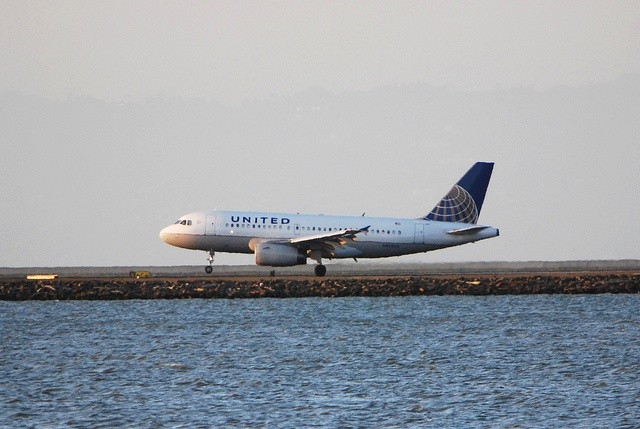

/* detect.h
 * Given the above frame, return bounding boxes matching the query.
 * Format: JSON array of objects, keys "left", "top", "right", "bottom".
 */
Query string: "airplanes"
[{"left": 159, "top": 162, "right": 500, "bottom": 277}]
